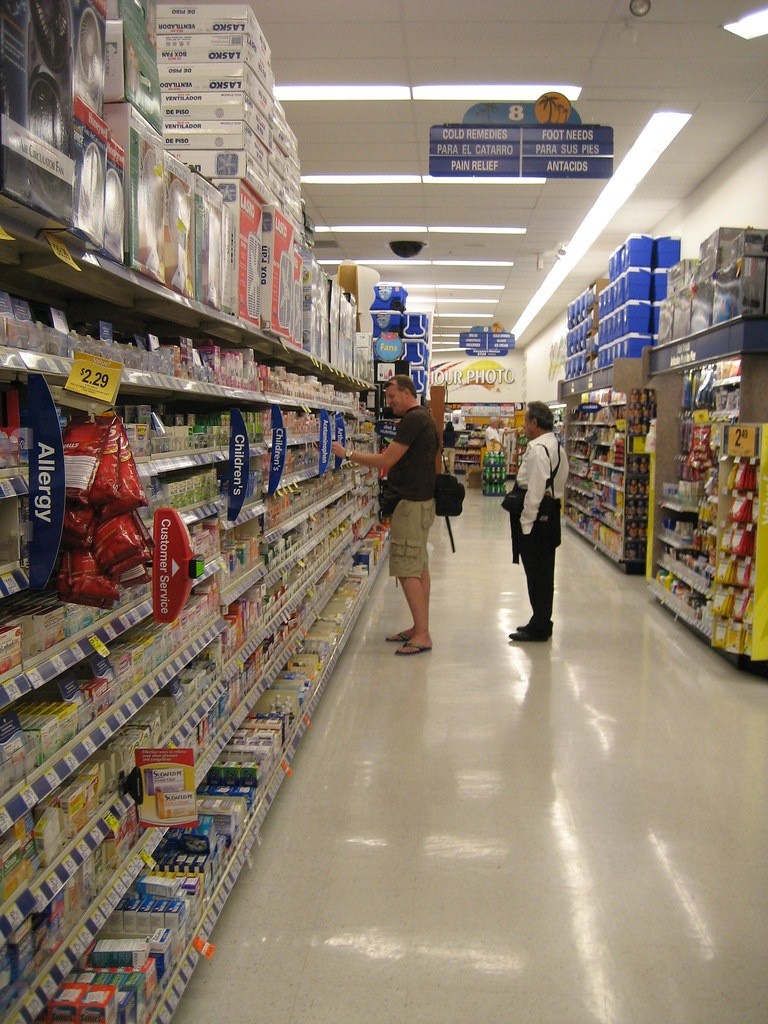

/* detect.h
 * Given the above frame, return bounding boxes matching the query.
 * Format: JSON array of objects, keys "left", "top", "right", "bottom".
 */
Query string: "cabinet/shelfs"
[{"left": 0, "top": 215, "right": 768, "bottom": 1024}]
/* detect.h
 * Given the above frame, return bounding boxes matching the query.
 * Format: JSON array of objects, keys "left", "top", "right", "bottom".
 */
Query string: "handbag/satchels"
[
  {"left": 502, "top": 488, "right": 527, "bottom": 512},
  {"left": 434, "top": 473, "right": 465, "bottom": 516},
  {"left": 529, "top": 496, "right": 562, "bottom": 552},
  {"left": 378, "top": 488, "right": 402, "bottom": 514}
]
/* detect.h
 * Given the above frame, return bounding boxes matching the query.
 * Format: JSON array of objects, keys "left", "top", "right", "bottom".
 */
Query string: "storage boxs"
[{"left": 0, "top": 0, "right": 353, "bottom": 374}]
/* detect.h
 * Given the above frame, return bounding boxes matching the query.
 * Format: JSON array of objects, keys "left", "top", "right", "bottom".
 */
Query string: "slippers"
[
  {"left": 395, "top": 643, "right": 432, "bottom": 655},
  {"left": 386, "top": 632, "right": 411, "bottom": 641}
]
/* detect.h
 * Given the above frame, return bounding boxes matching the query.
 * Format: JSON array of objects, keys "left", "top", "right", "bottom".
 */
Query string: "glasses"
[{"left": 384, "top": 382, "right": 406, "bottom": 390}]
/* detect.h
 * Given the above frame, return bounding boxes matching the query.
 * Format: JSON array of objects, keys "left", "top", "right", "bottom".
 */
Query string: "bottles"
[
  {"left": 482, "top": 467, "right": 507, "bottom": 484},
  {"left": 484, "top": 451, "right": 505, "bottom": 467},
  {"left": 482, "top": 479, "right": 507, "bottom": 496}
]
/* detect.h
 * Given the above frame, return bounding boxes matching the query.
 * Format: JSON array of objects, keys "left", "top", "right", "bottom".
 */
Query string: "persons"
[
  {"left": 508, "top": 401, "right": 570, "bottom": 642},
  {"left": 483, "top": 416, "right": 521, "bottom": 454},
  {"left": 330, "top": 375, "right": 441, "bottom": 656},
  {"left": 441, "top": 422, "right": 458, "bottom": 477}
]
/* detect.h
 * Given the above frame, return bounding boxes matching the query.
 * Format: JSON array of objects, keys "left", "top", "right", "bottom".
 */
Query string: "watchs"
[{"left": 345, "top": 449, "right": 353, "bottom": 461}]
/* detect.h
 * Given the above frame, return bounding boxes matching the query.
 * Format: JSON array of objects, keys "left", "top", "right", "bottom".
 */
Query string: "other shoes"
[
  {"left": 509, "top": 631, "right": 548, "bottom": 641},
  {"left": 517, "top": 627, "right": 552, "bottom": 636}
]
